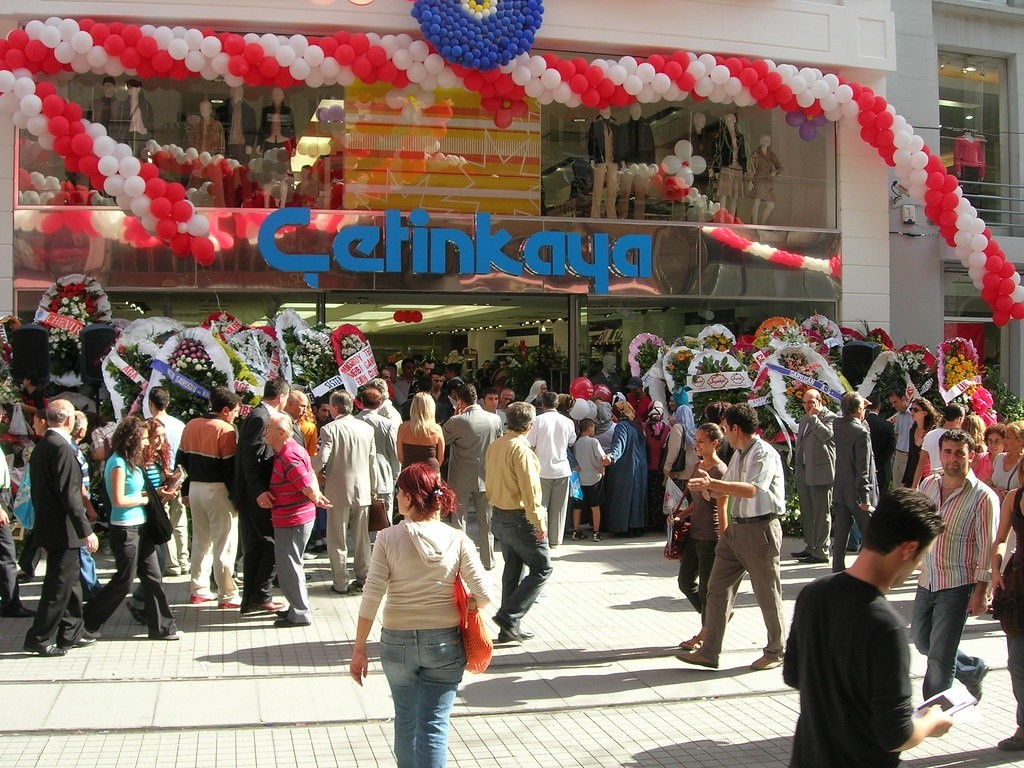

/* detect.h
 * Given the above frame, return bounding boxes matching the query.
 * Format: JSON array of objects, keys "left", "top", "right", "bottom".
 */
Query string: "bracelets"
[{"left": 990, "top": 571, "right": 1002, "bottom": 577}]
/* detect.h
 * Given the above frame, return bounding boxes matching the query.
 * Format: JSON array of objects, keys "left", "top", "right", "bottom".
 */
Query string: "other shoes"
[
  {"left": 274, "top": 619, "right": 311, "bottom": 627},
  {"left": 498, "top": 630, "right": 535, "bottom": 641},
  {"left": 492, "top": 616, "right": 525, "bottom": 644},
  {"left": 275, "top": 611, "right": 290, "bottom": 618},
  {"left": 0, "top": 604, "right": 36, "bottom": 617},
  {"left": 241, "top": 601, "right": 286, "bottom": 616},
  {"left": 352, "top": 580, "right": 363, "bottom": 590},
  {"left": 968, "top": 663, "right": 991, "bottom": 706},
  {"left": 331, "top": 584, "right": 347, "bottom": 594},
  {"left": 233, "top": 576, "right": 244, "bottom": 585},
  {"left": 23, "top": 638, "right": 68, "bottom": 657},
  {"left": 84, "top": 627, "right": 102, "bottom": 638},
  {"left": 163, "top": 630, "right": 185, "bottom": 640},
  {"left": 791, "top": 548, "right": 809, "bottom": 557},
  {"left": 218, "top": 597, "right": 242, "bottom": 609},
  {"left": 189, "top": 595, "right": 218, "bottom": 604},
  {"left": 680, "top": 630, "right": 704, "bottom": 650},
  {"left": 57, "top": 638, "right": 96, "bottom": 650},
  {"left": 125, "top": 601, "right": 147, "bottom": 625},
  {"left": 998, "top": 727, "right": 1024, "bottom": 750},
  {"left": 798, "top": 556, "right": 829, "bottom": 563}
]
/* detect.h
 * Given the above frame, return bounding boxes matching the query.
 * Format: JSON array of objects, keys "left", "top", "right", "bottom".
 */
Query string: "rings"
[{"left": 260, "top": 503, "right": 264, "bottom": 505}]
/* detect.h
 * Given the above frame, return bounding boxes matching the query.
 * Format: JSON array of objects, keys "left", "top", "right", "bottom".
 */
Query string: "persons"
[
  {"left": 87, "top": 77, "right": 155, "bottom": 203},
  {"left": 618, "top": 103, "right": 656, "bottom": 219},
  {"left": 783, "top": 487, "right": 954, "bottom": 768},
  {"left": 0, "top": 355, "right": 1024, "bottom": 752},
  {"left": 714, "top": 111, "right": 748, "bottom": 218},
  {"left": 257, "top": 86, "right": 296, "bottom": 177},
  {"left": 215, "top": 84, "right": 256, "bottom": 208},
  {"left": 351, "top": 463, "right": 494, "bottom": 768},
  {"left": 588, "top": 354, "right": 622, "bottom": 406},
  {"left": 261, "top": 116, "right": 292, "bottom": 208},
  {"left": 679, "top": 111, "right": 713, "bottom": 221},
  {"left": 295, "top": 164, "right": 319, "bottom": 199},
  {"left": 953, "top": 129, "right": 984, "bottom": 218},
  {"left": 587, "top": 102, "right": 621, "bottom": 221},
  {"left": 747, "top": 132, "right": 784, "bottom": 225},
  {"left": 182, "top": 100, "right": 225, "bottom": 200}
]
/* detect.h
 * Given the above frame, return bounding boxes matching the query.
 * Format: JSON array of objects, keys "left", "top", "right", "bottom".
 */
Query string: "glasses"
[{"left": 908, "top": 407, "right": 923, "bottom": 412}]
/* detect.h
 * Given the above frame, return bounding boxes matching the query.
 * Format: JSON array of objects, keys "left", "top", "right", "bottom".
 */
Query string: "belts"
[{"left": 731, "top": 512, "right": 775, "bottom": 523}]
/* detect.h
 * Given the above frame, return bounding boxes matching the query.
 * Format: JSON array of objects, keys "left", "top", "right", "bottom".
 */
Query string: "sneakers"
[
  {"left": 592, "top": 530, "right": 603, "bottom": 541},
  {"left": 750, "top": 655, "right": 784, "bottom": 669},
  {"left": 571, "top": 529, "right": 588, "bottom": 541},
  {"left": 676, "top": 651, "right": 718, "bottom": 669}
]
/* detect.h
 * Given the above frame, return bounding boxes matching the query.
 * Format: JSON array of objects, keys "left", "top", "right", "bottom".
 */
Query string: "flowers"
[
  {"left": 635, "top": 307, "right": 990, "bottom": 439},
  {"left": 0, "top": 270, "right": 368, "bottom": 431}
]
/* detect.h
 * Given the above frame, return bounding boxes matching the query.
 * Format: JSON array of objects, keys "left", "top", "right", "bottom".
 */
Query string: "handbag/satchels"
[
  {"left": 663, "top": 518, "right": 692, "bottom": 562},
  {"left": 454, "top": 577, "right": 493, "bottom": 673},
  {"left": 923, "top": 458, "right": 930, "bottom": 478},
  {"left": 369, "top": 500, "right": 391, "bottom": 532}
]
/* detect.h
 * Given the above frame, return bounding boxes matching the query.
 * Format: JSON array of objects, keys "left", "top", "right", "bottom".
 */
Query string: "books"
[{"left": 156, "top": 464, "right": 188, "bottom": 508}]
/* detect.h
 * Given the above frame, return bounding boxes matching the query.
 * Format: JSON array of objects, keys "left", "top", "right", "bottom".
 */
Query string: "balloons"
[
  {"left": 1, "top": 0, "right": 1024, "bottom": 326},
  {"left": 569, "top": 377, "right": 595, "bottom": 401},
  {"left": 591, "top": 384, "right": 612, "bottom": 403}
]
